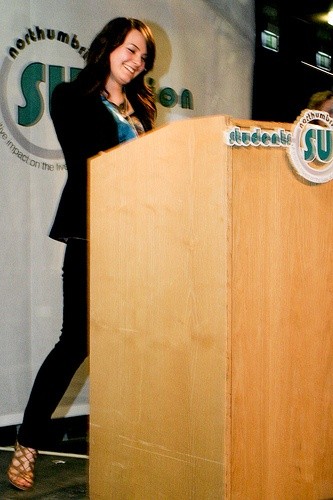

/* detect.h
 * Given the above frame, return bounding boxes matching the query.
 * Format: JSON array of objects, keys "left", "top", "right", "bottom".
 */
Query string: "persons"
[
  {"left": 7, "top": 17, "right": 157, "bottom": 492},
  {"left": 310, "top": 90, "right": 333, "bottom": 127}
]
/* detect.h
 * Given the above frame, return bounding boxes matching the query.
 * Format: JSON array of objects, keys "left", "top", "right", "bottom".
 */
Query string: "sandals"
[{"left": 7, "top": 440, "right": 38, "bottom": 491}]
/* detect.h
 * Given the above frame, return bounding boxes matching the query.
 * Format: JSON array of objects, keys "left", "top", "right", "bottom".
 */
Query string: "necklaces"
[{"left": 106, "top": 94, "right": 131, "bottom": 119}]
